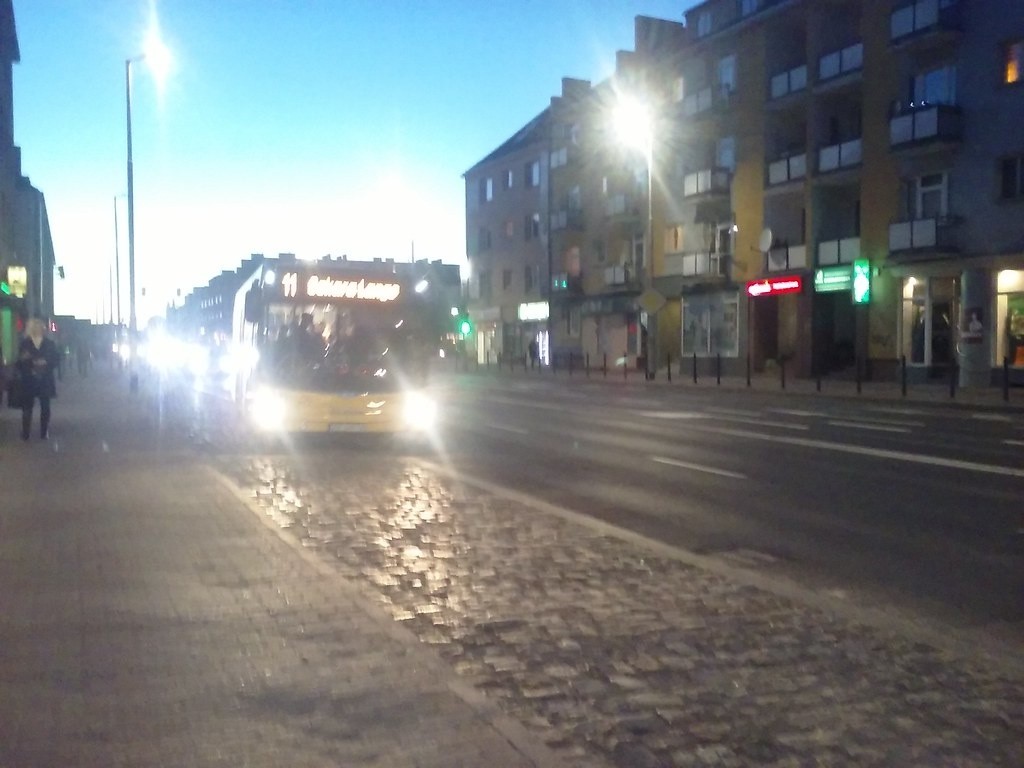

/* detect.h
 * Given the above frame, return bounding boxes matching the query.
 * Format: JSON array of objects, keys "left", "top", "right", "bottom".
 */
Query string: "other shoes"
[
  {"left": 20, "top": 432, "right": 30, "bottom": 440},
  {"left": 40, "top": 430, "right": 49, "bottom": 440}
]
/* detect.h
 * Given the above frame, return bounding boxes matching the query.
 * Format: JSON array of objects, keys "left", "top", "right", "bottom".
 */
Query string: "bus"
[{"left": 230, "top": 262, "right": 444, "bottom": 436}]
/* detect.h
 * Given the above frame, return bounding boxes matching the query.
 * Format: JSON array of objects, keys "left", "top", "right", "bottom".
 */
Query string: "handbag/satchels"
[{"left": 8, "top": 359, "right": 35, "bottom": 408}]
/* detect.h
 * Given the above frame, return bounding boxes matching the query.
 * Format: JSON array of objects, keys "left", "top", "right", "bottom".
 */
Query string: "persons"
[
  {"left": 969, "top": 312, "right": 983, "bottom": 332},
  {"left": 912, "top": 310, "right": 924, "bottom": 364},
  {"left": 269, "top": 312, "right": 327, "bottom": 390},
  {"left": 14, "top": 318, "right": 60, "bottom": 440},
  {"left": 77, "top": 342, "right": 90, "bottom": 380},
  {"left": 528, "top": 338, "right": 538, "bottom": 371}
]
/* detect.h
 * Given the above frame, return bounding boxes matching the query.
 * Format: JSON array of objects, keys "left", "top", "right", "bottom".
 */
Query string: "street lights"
[{"left": 123, "top": 33, "right": 164, "bottom": 400}]
